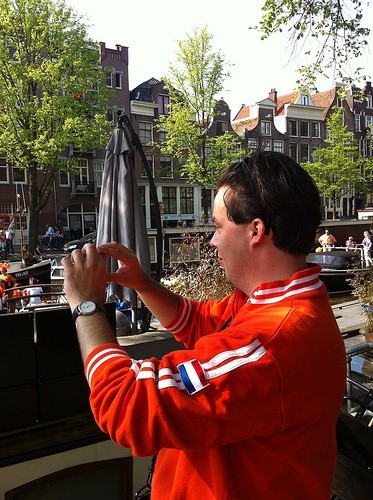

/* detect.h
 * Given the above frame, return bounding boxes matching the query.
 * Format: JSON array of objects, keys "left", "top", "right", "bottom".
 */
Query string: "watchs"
[{"left": 70, "top": 299, "right": 107, "bottom": 335}]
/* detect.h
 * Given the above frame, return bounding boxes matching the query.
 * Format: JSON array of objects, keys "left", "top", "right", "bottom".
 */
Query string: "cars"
[
  {"left": 305, "top": 251, "right": 361, "bottom": 298},
  {"left": 63, "top": 231, "right": 97, "bottom": 252}
]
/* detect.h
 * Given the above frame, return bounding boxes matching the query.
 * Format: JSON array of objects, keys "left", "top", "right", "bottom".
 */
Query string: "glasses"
[{"left": 232, "top": 157, "right": 258, "bottom": 191}]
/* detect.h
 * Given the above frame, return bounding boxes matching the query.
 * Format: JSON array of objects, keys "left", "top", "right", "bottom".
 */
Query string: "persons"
[
  {"left": 0, "top": 262, "right": 48, "bottom": 314},
  {"left": 61, "top": 152, "right": 346, "bottom": 500},
  {"left": 317, "top": 227, "right": 373, "bottom": 269},
  {"left": 41, "top": 224, "right": 64, "bottom": 250},
  {"left": 113, "top": 294, "right": 141, "bottom": 321},
  {"left": 0, "top": 225, "right": 15, "bottom": 262}
]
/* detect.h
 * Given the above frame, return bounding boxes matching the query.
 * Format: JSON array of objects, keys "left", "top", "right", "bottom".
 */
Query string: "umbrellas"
[{"left": 96, "top": 127, "right": 152, "bottom": 333}]
